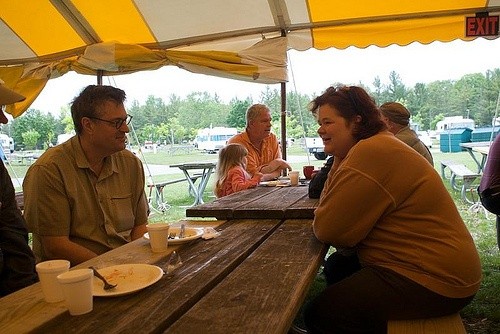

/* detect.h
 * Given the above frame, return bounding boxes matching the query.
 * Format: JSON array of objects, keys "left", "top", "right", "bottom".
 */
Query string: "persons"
[
  {"left": 379, "top": 102, "right": 434, "bottom": 167},
  {"left": 214, "top": 143, "right": 263, "bottom": 198},
  {"left": 22, "top": 85, "right": 148, "bottom": 267},
  {"left": 0, "top": 107, "right": 40, "bottom": 299},
  {"left": 226, "top": 104, "right": 292, "bottom": 181},
  {"left": 477, "top": 133, "right": 500, "bottom": 248},
  {"left": 285, "top": 85, "right": 482, "bottom": 334}
]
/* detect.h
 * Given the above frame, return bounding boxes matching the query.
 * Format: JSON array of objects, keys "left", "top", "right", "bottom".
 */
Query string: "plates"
[
  {"left": 91, "top": 263, "right": 163, "bottom": 297},
  {"left": 143, "top": 227, "right": 203, "bottom": 244}
]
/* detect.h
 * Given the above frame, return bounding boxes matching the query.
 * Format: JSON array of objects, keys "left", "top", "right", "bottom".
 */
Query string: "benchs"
[
  {"left": 147, "top": 171, "right": 217, "bottom": 211},
  {"left": 386, "top": 312, "right": 468, "bottom": 334},
  {"left": 440, "top": 160, "right": 481, "bottom": 203}
]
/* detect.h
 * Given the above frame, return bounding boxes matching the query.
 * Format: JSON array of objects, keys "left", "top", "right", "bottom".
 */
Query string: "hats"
[{"left": 380, "top": 101, "right": 411, "bottom": 125}]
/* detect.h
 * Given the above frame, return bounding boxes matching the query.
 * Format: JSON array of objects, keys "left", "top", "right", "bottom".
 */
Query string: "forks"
[{"left": 88, "top": 266, "right": 118, "bottom": 290}]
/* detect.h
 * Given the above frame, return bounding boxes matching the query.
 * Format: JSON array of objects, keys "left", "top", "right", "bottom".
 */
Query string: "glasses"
[{"left": 88, "top": 114, "right": 134, "bottom": 130}]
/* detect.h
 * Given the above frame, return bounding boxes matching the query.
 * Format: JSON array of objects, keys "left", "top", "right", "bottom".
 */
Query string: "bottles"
[{"left": 311, "top": 170, "right": 318, "bottom": 179}]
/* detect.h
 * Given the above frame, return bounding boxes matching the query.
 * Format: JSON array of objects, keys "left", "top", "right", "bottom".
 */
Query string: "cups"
[
  {"left": 289, "top": 171, "right": 299, "bottom": 186},
  {"left": 57, "top": 268, "right": 94, "bottom": 315},
  {"left": 35, "top": 259, "right": 71, "bottom": 303},
  {"left": 146, "top": 223, "right": 170, "bottom": 252},
  {"left": 303, "top": 166, "right": 314, "bottom": 180}
]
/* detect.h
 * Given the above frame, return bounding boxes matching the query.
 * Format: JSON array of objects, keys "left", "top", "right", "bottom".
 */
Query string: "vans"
[
  {"left": 194, "top": 126, "right": 238, "bottom": 154},
  {"left": 0, "top": 134, "right": 14, "bottom": 154}
]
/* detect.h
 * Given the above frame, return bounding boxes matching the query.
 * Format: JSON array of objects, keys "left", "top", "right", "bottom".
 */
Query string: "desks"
[
  {"left": 458, "top": 142, "right": 491, "bottom": 172},
  {"left": 0, "top": 216, "right": 332, "bottom": 334},
  {"left": 473, "top": 146, "right": 492, "bottom": 172},
  {"left": 184, "top": 173, "right": 320, "bottom": 219},
  {"left": 169, "top": 160, "right": 219, "bottom": 205}
]
